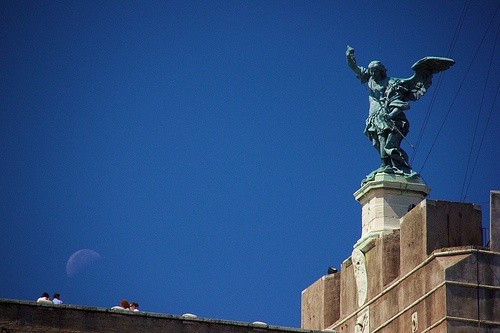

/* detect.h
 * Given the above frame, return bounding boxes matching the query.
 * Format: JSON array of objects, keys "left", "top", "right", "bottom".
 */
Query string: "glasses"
[{"left": 129, "top": 306, "right": 132, "bottom": 307}]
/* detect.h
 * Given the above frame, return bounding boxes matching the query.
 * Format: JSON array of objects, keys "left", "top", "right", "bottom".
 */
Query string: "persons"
[
  {"left": 346, "top": 45, "right": 456, "bottom": 173},
  {"left": 37, "top": 292, "right": 53, "bottom": 304},
  {"left": 110, "top": 300, "right": 129, "bottom": 310},
  {"left": 129, "top": 302, "right": 139, "bottom": 311},
  {"left": 52, "top": 293, "right": 63, "bottom": 304}
]
